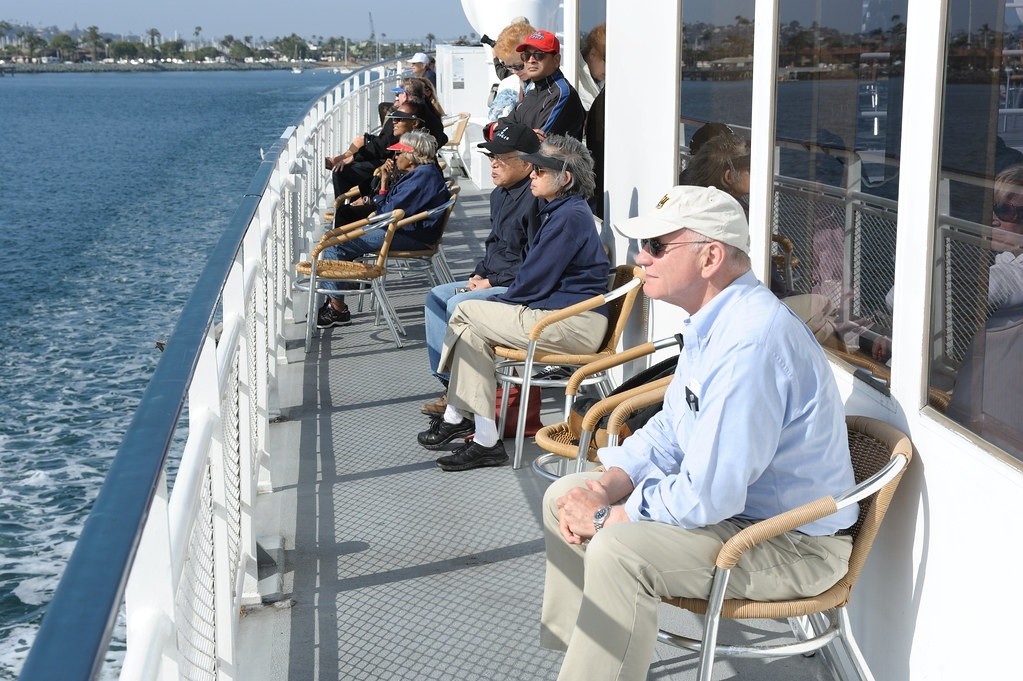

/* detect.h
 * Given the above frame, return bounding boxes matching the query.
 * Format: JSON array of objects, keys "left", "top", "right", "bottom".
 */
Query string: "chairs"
[
  {"left": 491, "top": 265, "right": 645, "bottom": 469},
  {"left": 294, "top": 112, "right": 471, "bottom": 353},
  {"left": 605, "top": 388, "right": 912, "bottom": 681},
  {"left": 530, "top": 293, "right": 831, "bottom": 483}
]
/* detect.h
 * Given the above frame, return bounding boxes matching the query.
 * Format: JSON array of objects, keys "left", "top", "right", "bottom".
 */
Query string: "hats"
[
  {"left": 389, "top": 87, "right": 411, "bottom": 94},
  {"left": 690, "top": 122, "right": 734, "bottom": 154},
  {"left": 387, "top": 142, "right": 418, "bottom": 153},
  {"left": 516, "top": 30, "right": 559, "bottom": 54},
  {"left": 520, "top": 152, "right": 574, "bottom": 172},
  {"left": 613, "top": 185, "right": 750, "bottom": 254},
  {"left": 406, "top": 53, "right": 429, "bottom": 64},
  {"left": 477, "top": 124, "right": 540, "bottom": 154},
  {"left": 385, "top": 110, "right": 425, "bottom": 123}
]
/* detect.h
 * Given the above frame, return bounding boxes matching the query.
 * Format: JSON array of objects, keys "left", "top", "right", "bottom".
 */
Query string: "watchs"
[{"left": 594, "top": 504, "right": 611, "bottom": 531}]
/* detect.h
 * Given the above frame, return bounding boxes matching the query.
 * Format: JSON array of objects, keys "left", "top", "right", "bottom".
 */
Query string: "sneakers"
[
  {"left": 421, "top": 391, "right": 472, "bottom": 418},
  {"left": 529, "top": 365, "right": 571, "bottom": 390},
  {"left": 436, "top": 439, "right": 509, "bottom": 471},
  {"left": 316, "top": 305, "right": 352, "bottom": 328},
  {"left": 417, "top": 414, "right": 476, "bottom": 449},
  {"left": 305, "top": 300, "right": 348, "bottom": 318}
]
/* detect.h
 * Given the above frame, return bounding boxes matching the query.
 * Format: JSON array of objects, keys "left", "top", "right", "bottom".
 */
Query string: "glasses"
[
  {"left": 488, "top": 154, "right": 521, "bottom": 162},
  {"left": 643, "top": 237, "right": 713, "bottom": 256},
  {"left": 531, "top": 165, "right": 563, "bottom": 176},
  {"left": 505, "top": 63, "right": 524, "bottom": 70},
  {"left": 520, "top": 50, "right": 556, "bottom": 62},
  {"left": 993, "top": 201, "right": 1023, "bottom": 222},
  {"left": 396, "top": 153, "right": 413, "bottom": 156},
  {"left": 394, "top": 118, "right": 414, "bottom": 122}
]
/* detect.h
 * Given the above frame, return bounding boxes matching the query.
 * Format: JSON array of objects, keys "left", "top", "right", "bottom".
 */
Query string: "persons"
[
  {"left": 576, "top": 26, "right": 606, "bottom": 111},
  {"left": 579, "top": 81, "right": 605, "bottom": 221},
  {"left": 679, "top": 122, "right": 852, "bottom": 353},
  {"left": 418, "top": 131, "right": 610, "bottom": 471},
  {"left": 870, "top": 64, "right": 1023, "bottom": 362},
  {"left": 485, "top": 18, "right": 585, "bottom": 143},
  {"left": 418, "top": 124, "right": 540, "bottom": 416},
  {"left": 305, "top": 49, "right": 450, "bottom": 328},
  {"left": 539, "top": 181, "right": 859, "bottom": 681}
]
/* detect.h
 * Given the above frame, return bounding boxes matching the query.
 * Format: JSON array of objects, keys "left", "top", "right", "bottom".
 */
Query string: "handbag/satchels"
[{"left": 495, "top": 367, "right": 546, "bottom": 437}]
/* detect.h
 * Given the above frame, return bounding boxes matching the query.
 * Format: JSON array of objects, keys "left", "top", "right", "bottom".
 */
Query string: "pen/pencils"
[{"left": 689, "top": 394, "right": 696, "bottom": 417}]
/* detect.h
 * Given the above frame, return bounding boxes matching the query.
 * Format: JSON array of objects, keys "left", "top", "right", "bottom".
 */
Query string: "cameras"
[{"left": 479, "top": 34, "right": 496, "bottom": 48}]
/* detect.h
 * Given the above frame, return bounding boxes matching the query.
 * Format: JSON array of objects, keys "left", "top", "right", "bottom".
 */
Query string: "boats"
[
  {"left": 338, "top": 65, "right": 353, "bottom": 75},
  {"left": 291, "top": 65, "right": 304, "bottom": 74}
]
